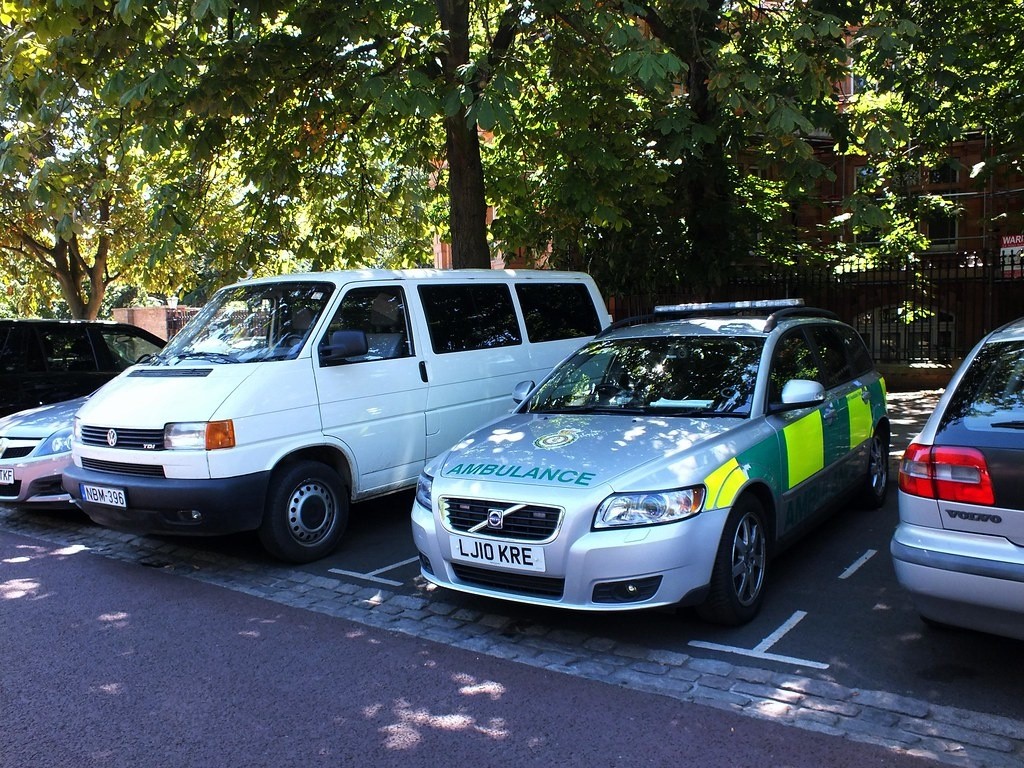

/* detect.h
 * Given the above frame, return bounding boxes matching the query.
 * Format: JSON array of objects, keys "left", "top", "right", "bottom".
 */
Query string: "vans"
[
  {"left": 62, "top": 263, "right": 612, "bottom": 567},
  {"left": 0, "top": 319, "right": 170, "bottom": 418}
]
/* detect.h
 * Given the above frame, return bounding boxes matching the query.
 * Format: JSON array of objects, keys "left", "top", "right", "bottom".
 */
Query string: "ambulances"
[{"left": 409, "top": 296, "right": 892, "bottom": 628}]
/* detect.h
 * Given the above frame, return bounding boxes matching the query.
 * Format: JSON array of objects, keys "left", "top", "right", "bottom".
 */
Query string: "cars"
[
  {"left": 0, "top": 382, "right": 111, "bottom": 512},
  {"left": 891, "top": 315, "right": 1024, "bottom": 644}
]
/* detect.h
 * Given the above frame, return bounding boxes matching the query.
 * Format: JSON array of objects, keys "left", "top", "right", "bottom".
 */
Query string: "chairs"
[
  {"left": 345, "top": 293, "right": 404, "bottom": 363},
  {"left": 282, "top": 305, "right": 315, "bottom": 349}
]
